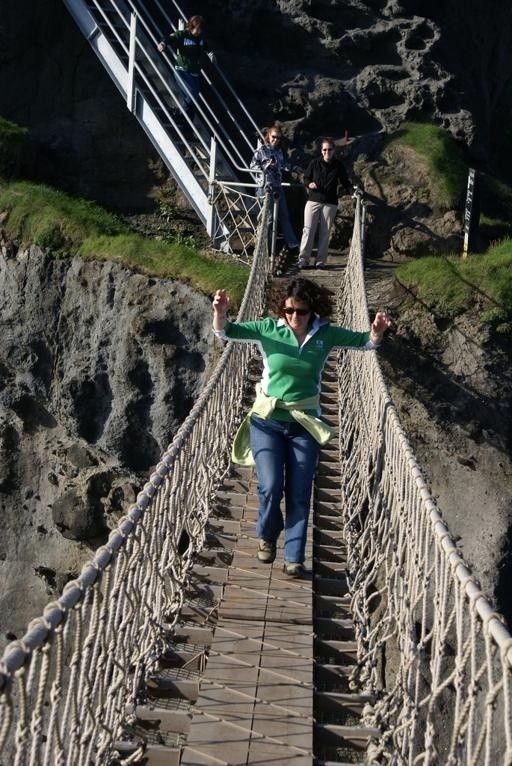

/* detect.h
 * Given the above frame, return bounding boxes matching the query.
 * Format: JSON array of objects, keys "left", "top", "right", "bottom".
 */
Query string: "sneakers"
[
  {"left": 298, "top": 261, "right": 308, "bottom": 269},
  {"left": 283, "top": 561, "right": 304, "bottom": 578},
  {"left": 315, "top": 261, "right": 324, "bottom": 269},
  {"left": 257, "top": 538, "right": 276, "bottom": 564}
]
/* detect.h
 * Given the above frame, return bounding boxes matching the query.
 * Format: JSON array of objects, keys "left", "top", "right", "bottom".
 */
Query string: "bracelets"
[
  {"left": 372, "top": 331, "right": 381, "bottom": 337},
  {"left": 214, "top": 312, "right": 226, "bottom": 318}
]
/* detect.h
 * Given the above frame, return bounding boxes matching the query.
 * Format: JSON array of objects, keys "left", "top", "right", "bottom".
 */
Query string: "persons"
[
  {"left": 212, "top": 277, "right": 393, "bottom": 575},
  {"left": 250, "top": 126, "right": 303, "bottom": 251},
  {"left": 296, "top": 137, "right": 363, "bottom": 269},
  {"left": 157, "top": 14, "right": 217, "bottom": 141}
]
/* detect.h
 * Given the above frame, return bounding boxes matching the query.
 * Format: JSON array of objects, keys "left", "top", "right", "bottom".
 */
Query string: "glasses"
[
  {"left": 282, "top": 308, "right": 311, "bottom": 315},
  {"left": 323, "top": 148, "right": 333, "bottom": 151},
  {"left": 271, "top": 136, "right": 280, "bottom": 139}
]
